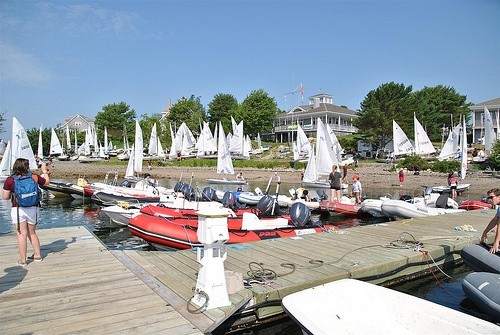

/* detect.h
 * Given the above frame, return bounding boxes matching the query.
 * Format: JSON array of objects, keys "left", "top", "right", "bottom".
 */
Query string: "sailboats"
[
  {"left": 37, "top": 116, "right": 270, "bottom": 163},
  {"left": 277, "top": 118, "right": 358, "bottom": 190},
  {"left": 392, "top": 106, "right": 500, "bottom": 179}
]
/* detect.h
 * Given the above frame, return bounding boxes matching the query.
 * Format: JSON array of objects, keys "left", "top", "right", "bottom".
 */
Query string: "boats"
[
  {"left": 461, "top": 271, "right": 500, "bottom": 323},
  {"left": 127, "top": 214, "right": 339, "bottom": 250},
  {"left": 460, "top": 242, "right": 500, "bottom": 275},
  {"left": 320, "top": 191, "right": 492, "bottom": 220},
  {"left": 44, "top": 170, "right": 322, "bottom": 231},
  {"left": 422, "top": 183, "right": 471, "bottom": 193},
  {"left": 282, "top": 278, "right": 500, "bottom": 335}
]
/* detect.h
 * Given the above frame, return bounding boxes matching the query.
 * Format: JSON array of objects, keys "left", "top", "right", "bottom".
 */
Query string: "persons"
[
  {"left": 472, "top": 148, "right": 485, "bottom": 158},
  {"left": 351, "top": 175, "right": 362, "bottom": 204},
  {"left": 458, "top": 149, "right": 461, "bottom": 159},
  {"left": 177, "top": 151, "right": 181, "bottom": 161},
  {"left": 448, "top": 169, "right": 457, "bottom": 199},
  {"left": 355, "top": 158, "right": 359, "bottom": 171},
  {"left": 399, "top": 168, "right": 404, "bottom": 186},
  {"left": 343, "top": 164, "right": 347, "bottom": 184},
  {"left": 148, "top": 160, "right": 152, "bottom": 170},
  {"left": 481, "top": 188, "right": 500, "bottom": 253},
  {"left": 3, "top": 158, "right": 49, "bottom": 266},
  {"left": 236, "top": 173, "right": 245, "bottom": 180},
  {"left": 329, "top": 165, "right": 342, "bottom": 202},
  {"left": 295, "top": 188, "right": 308, "bottom": 201}
]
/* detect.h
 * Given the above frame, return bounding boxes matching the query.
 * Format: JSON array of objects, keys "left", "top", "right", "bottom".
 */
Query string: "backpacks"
[{"left": 10, "top": 173, "right": 40, "bottom": 207}]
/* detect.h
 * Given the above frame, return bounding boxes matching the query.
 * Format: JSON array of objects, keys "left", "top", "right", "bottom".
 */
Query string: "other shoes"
[
  {"left": 27, "top": 254, "right": 44, "bottom": 262},
  {"left": 16, "top": 259, "right": 29, "bottom": 267}
]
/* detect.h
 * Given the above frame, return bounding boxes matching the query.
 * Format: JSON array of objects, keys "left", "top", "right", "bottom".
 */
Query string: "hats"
[{"left": 352, "top": 175, "right": 359, "bottom": 178}]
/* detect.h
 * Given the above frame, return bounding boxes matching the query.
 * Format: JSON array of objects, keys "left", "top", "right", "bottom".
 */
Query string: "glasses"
[{"left": 487, "top": 195, "right": 497, "bottom": 199}]
